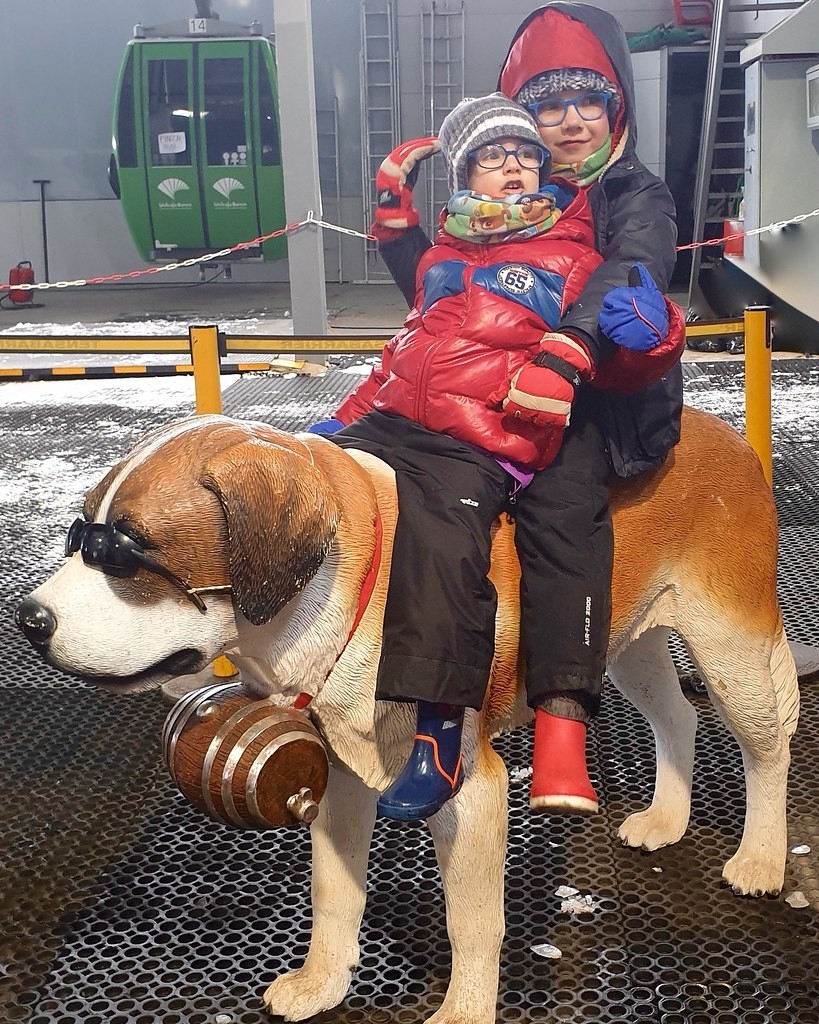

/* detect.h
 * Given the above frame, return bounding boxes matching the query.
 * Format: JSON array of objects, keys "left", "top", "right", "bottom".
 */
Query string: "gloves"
[
  {"left": 486, "top": 332, "right": 596, "bottom": 433},
  {"left": 599, "top": 262, "right": 670, "bottom": 352},
  {"left": 306, "top": 419, "right": 344, "bottom": 434},
  {"left": 375, "top": 137, "right": 439, "bottom": 229}
]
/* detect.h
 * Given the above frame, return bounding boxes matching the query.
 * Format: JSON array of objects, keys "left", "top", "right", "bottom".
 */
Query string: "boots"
[
  {"left": 528, "top": 708, "right": 599, "bottom": 816},
  {"left": 377, "top": 702, "right": 465, "bottom": 820}
]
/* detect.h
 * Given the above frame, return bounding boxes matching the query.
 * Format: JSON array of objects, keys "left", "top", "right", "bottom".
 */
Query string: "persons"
[
  {"left": 368, "top": 0, "right": 684, "bottom": 815},
  {"left": 307, "top": 92, "right": 687, "bottom": 817}
]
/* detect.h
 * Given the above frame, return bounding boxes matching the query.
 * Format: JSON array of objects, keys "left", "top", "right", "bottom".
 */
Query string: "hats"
[
  {"left": 514, "top": 69, "right": 621, "bottom": 131},
  {"left": 437, "top": 91, "right": 553, "bottom": 196}
]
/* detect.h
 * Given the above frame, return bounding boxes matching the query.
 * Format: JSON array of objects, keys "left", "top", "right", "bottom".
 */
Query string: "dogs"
[{"left": 13, "top": 399, "right": 800, "bottom": 1024}]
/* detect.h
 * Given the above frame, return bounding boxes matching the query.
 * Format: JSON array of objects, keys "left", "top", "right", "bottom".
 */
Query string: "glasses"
[
  {"left": 62, "top": 518, "right": 207, "bottom": 611},
  {"left": 467, "top": 143, "right": 550, "bottom": 169},
  {"left": 524, "top": 91, "right": 612, "bottom": 127}
]
